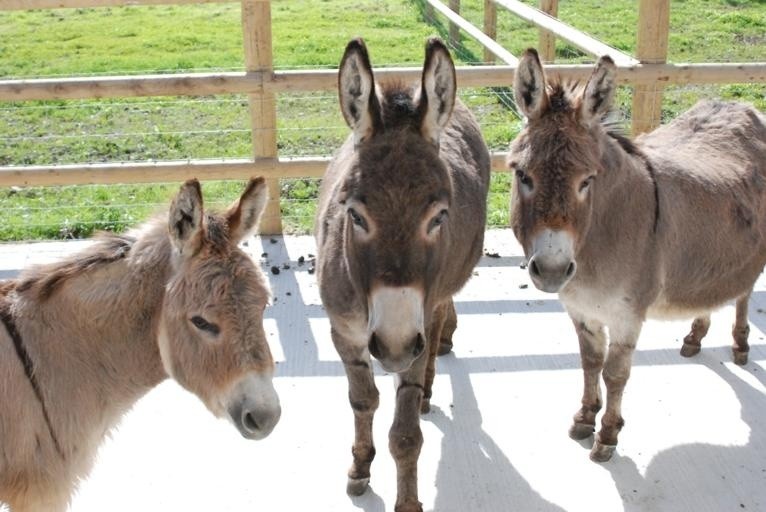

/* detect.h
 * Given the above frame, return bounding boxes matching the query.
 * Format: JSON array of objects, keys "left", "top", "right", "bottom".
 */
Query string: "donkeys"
[
  {"left": 304, "top": 33, "right": 494, "bottom": 511},
  {"left": 0, "top": 172, "right": 285, "bottom": 512},
  {"left": 503, "top": 45, "right": 766, "bottom": 464}
]
